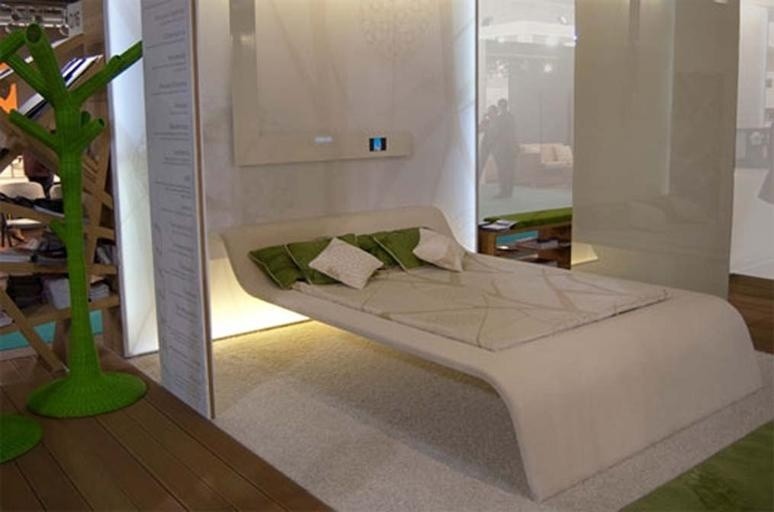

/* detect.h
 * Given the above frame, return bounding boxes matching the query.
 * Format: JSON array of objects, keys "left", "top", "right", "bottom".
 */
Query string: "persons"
[
  {"left": 23, "top": 150, "right": 54, "bottom": 199},
  {"left": 479, "top": 98, "right": 520, "bottom": 199}
]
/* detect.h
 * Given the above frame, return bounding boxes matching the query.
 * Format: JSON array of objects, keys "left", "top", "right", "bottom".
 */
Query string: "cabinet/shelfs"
[
  {"left": 475, "top": 218, "right": 572, "bottom": 271},
  {"left": 0, "top": 33, "right": 125, "bottom": 378}
]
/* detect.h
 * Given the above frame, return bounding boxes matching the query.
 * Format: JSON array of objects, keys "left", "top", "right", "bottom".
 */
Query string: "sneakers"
[{"left": 492, "top": 190, "right": 514, "bottom": 201}]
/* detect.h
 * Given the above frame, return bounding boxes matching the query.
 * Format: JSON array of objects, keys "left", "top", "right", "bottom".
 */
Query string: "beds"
[{"left": 216, "top": 204, "right": 768, "bottom": 505}]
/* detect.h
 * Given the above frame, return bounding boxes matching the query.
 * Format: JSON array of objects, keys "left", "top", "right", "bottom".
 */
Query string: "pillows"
[
  {"left": 369, "top": 225, "right": 427, "bottom": 274},
  {"left": 307, "top": 236, "right": 386, "bottom": 290},
  {"left": 356, "top": 230, "right": 395, "bottom": 267},
  {"left": 283, "top": 232, "right": 360, "bottom": 286},
  {"left": 246, "top": 242, "right": 303, "bottom": 290},
  {"left": 411, "top": 226, "right": 467, "bottom": 274}
]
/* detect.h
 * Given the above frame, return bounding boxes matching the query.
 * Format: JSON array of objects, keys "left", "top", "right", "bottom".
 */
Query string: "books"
[
  {"left": 0, "top": 239, "right": 112, "bottom": 265},
  {"left": 517, "top": 238, "right": 558, "bottom": 249}
]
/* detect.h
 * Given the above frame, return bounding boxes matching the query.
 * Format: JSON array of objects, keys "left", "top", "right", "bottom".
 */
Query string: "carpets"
[
  {"left": 622, "top": 419, "right": 773, "bottom": 512},
  {"left": 129, "top": 318, "right": 774, "bottom": 510}
]
